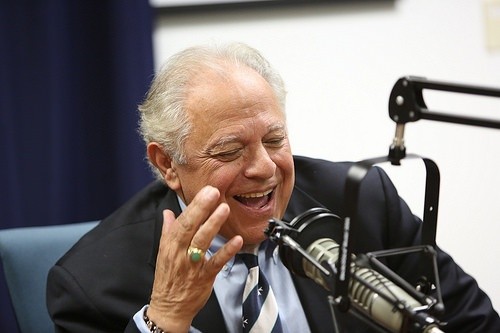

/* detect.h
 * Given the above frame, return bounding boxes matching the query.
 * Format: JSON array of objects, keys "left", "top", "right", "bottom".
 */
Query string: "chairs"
[{"left": 0, "top": 220, "right": 108, "bottom": 333}]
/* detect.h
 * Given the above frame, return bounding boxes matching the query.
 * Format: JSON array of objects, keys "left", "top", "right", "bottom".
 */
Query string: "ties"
[{"left": 234, "top": 241, "right": 284, "bottom": 333}]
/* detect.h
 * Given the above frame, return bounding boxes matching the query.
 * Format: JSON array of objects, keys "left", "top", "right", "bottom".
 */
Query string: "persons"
[{"left": 43, "top": 40, "right": 500, "bottom": 333}]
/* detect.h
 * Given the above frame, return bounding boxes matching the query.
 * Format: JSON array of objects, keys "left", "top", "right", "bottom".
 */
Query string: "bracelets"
[{"left": 140, "top": 311, "right": 169, "bottom": 333}]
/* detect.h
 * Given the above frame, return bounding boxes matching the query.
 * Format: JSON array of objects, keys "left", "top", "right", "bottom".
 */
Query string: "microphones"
[{"left": 265, "top": 207, "right": 446, "bottom": 333}]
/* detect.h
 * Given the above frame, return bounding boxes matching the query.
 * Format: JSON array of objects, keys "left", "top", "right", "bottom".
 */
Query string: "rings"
[{"left": 185, "top": 245, "right": 206, "bottom": 264}]
